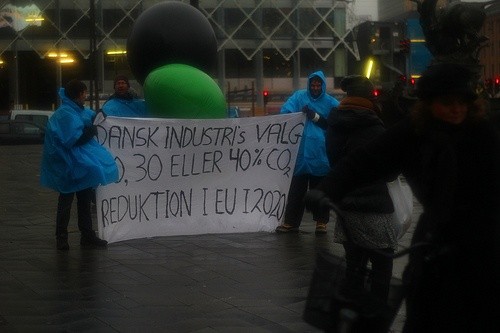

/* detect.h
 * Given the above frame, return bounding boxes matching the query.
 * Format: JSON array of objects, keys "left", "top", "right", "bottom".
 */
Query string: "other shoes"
[
  {"left": 316, "top": 223, "right": 327, "bottom": 233},
  {"left": 55, "top": 239, "right": 69, "bottom": 251},
  {"left": 80, "top": 232, "right": 108, "bottom": 247},
  {"left": 277, "top": 222, "right": 299, "bottom": 232}
]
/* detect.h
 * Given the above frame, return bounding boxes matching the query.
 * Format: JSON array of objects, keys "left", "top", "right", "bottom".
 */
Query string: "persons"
[
  {"left": 301, "top": 76, "right": 500, "bottom": 333},
  {"left": 324, "top": 74, "right": 398, "bottom": 333},
  {"left": 275, "top": 71, "right": 340, "bottom": 233},
  {"left": 101, "top": 77, "right": 147, "bottom": 118},
  {"left": 37, "top": 80, "right": 119, "bottom": 251}
]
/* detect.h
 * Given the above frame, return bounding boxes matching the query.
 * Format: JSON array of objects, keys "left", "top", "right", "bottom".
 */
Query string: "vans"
[{"left": 8, "top": 110, "right": 55, "bottom": 136}]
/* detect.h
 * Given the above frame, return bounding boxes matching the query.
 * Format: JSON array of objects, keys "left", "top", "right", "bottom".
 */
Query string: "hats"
[
  {"left": 340, "top": 76, "right": 375, "bottom": 99},
  {"left": 414, "top": 63, "right": 478, "bottom": 105}
]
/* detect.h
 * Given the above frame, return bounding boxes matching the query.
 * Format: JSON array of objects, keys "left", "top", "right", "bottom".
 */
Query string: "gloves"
[
  {"left": 86, "top": 125, "right": 98, "bottom": 136},
  {"left": 302, "top": 103, "right": 314, "bottom": 119},
  {"left": 96, "top": 109, "right": 106, "bottom": 121},
  {"left": 126, "top": 87, "right": 140, "bottom": 98}
]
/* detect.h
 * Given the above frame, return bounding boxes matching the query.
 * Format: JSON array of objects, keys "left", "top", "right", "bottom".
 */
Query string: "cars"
[{"left": 0, "top": 121, "right": 43, "bottom": 145}]
